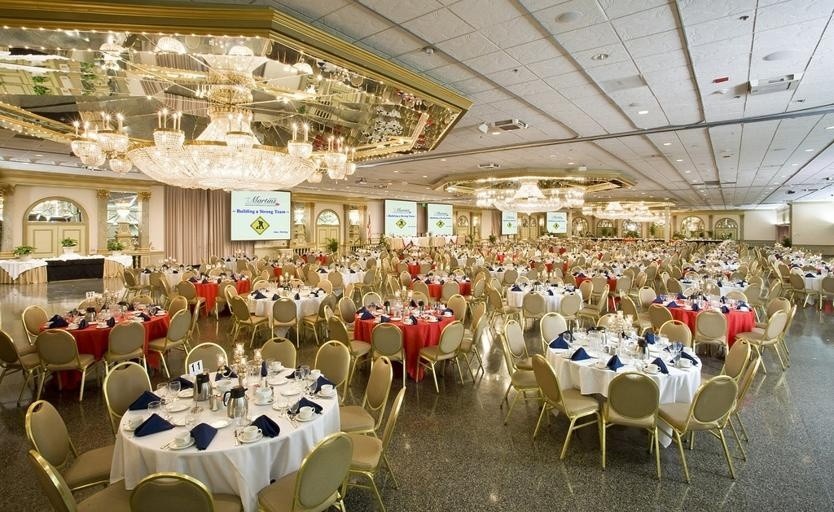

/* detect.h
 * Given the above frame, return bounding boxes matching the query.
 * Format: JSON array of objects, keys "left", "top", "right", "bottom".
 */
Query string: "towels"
[
  {"left": 285, "top": 365, "right": 311, "bottom": 380},
  {"left": 307, "top": 376, "right": 337, "bottom": 393},
  {"left": 190, "top": 423, "right": 218, "bottom": 451},
  {"left": 251, "top": 415, "right": 280, "bottom": 439},
  {"left": 571, "top": 347, "right": 596, "bottom": 361},
  {"left": 668, "top": 351, "right": 699, "bottom": 367},
  {"left": 165, "top": 376, "right": 193, "bottom": 392},
  {"left": 288, "top": 397, "right": 324, "bottom": 417},
  {"left": 129, "top": 391, "right": 165, "bottom": 410},
  {"left": 136, "top": 414, "right": 176, "bottom": 437},
  {"left": 261, "top": 361, "right": 267, "bottom": 377},
  {"left": 607, "top": 354, "right": 623, "bottom": 371},
  {"left": 215, "top": 365, "right": 238, "bottom": 380},
  {"left": 649, "top": 358, "right": 669, "bottom": 374}
]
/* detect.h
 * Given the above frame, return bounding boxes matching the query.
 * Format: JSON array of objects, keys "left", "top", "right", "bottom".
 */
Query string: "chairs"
[
  {"left": 24, "top": 400, "right": 115, "bottom": 493},
  {"left": 531, "top": 353, "right": 604, "bottom": 460},
  {"left": 184, "top": 342, "right": 228, "bottom": 376},
  {"left": 315, "top": 340, "right": 351, "bottom": 407},
  {"left": 460, "top": 302, "right": 485, "bottom": 384},
  {"left": 370, "top": 322, "right": 408, "bottom": 388},
  {"left": 599, "top": 371, "right": 662, "bottom": 479},
  {"left": 260, "top": 337, "right": 297, "bottom": 369},
  {"left": 327, "top": 316, "right": 371, "bottom": 386},
  {"left": 148, "top": 309, "right": 192, "bottom": 380},
  {"left": 256, "top": 432, "right": 354, "bottom": 512},
  {"left": 415, "top": 319, "right": 465, "bottom": 395},
  {"left": 130, "top": 471, "right": 243, "bottom": 512},
  {"left": 33, "top": 329, "right": 103, "bottom": 403},
  {"left": 102, "top": 361, "right": 153, "bottom": 441},
  {"left": 659, "top": 375, "right": 738, "bottom": 485},
  {"left": 0, "top": 330, "right": 60, "bottom": 404},
  {"left": 689, "top": 337, "right": 752, "bottom": 463},
  {"left": 27, "top": 449, "right": 133, "bottom": 512},
  {"left": 500, "top": 335, "right": 551, "bottom": 426},
  {"left": 103, "top": 321, "right": 148, "bottom": 384},
  {"left": 338, "top": 386, "right": 407, "bottom": 512},
  {"left": 338, "top": 355, "right": 394, "bottom": 439}
]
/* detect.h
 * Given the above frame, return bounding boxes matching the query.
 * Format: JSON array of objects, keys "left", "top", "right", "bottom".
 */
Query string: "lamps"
[
  {"left": 588, "top": 199, "right": 663, "bottom": 223},
  {"left": 447, "top": 178, "right": 584, "bottom": 215},
  {"left": 55, "top": 50, "right": 356, "bottom": 191}
]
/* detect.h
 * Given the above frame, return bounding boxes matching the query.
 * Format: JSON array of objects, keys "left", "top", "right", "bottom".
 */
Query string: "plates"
[
  {"left": 659, "top": 291, "right": 738, "bottom": 313},
  {"left": 45, "top": 305, "right": 165, "bottom": 331},
  {"left": 121, "top": 343, "right": 336, "bottom": 452},
  {"left": 252, "top": 283, "right": 325, "bottom": 301},
  {"left": 512, "top": 280, "right": 575, "bottom": 295},
  {"left": 563, "top": 326, "right": 698, "bottom": 375},
  {"left": 358, "top": 291, "right": 453, "bottom": 325}
]
